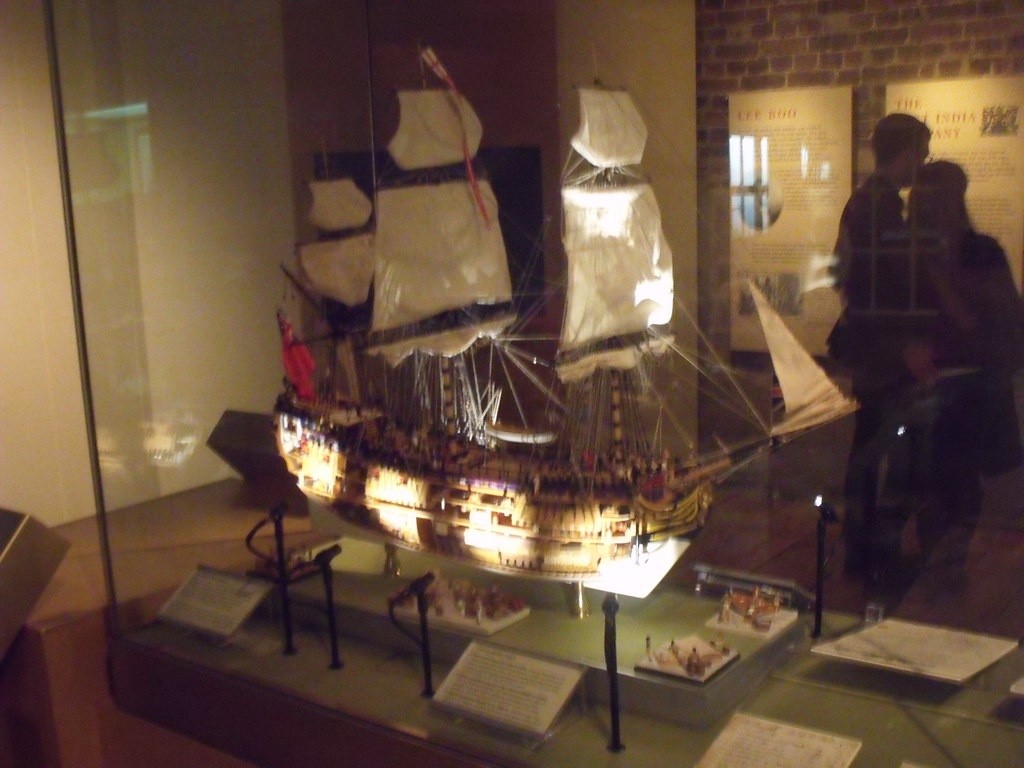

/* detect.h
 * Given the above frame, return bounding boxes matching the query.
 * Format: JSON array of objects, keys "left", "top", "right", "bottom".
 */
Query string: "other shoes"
[{"left": 842, "top": 545, "right": 908, "bottom": 591}]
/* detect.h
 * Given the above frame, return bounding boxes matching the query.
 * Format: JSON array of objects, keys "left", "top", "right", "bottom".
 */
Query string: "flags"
[{"left": 278, "top": 314, "right": 314, "bottom": 399}]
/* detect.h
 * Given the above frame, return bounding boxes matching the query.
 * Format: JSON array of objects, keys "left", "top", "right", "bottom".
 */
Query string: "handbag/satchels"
[{"left": 826, "top": 303, "right": 894, "bottom": 372}]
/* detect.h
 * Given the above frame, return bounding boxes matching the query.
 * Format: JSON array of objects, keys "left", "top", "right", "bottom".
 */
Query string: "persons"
[
  {"left": 829, "top": 113, "right": 1024, "bottom": 592},
  {"left": 646, "top": 637, "right": 701, "bottom": 668},
  {"left": 429, "top": 577, "right": 497, "bottom": 623},
  {"left": 719, "top": 586, "right": 780, "bottom": 622},
  {"left": 268, "top": 545, "right": 313, "bottom": 568}
]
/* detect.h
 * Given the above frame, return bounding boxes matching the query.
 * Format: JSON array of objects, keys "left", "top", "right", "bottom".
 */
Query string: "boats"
[{"left": 274, "top": 43, "right": 858, "bottom": 576}]
